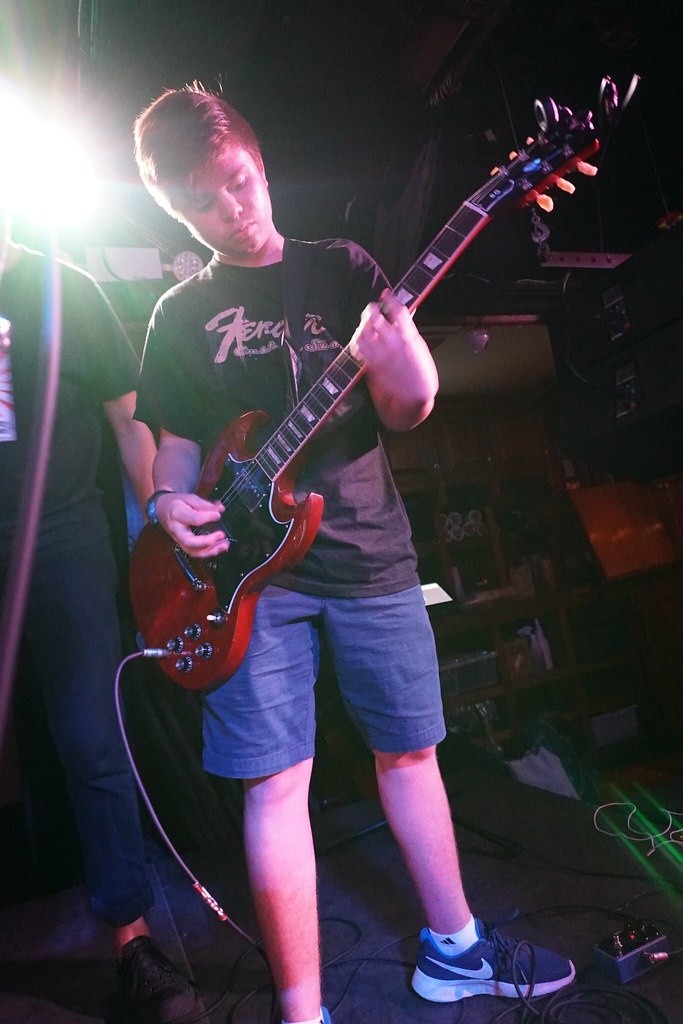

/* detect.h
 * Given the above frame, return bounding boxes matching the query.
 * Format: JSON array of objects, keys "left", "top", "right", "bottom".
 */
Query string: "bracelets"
[{"left": 145, "top": 490, "right": 172, "bottom": 525}]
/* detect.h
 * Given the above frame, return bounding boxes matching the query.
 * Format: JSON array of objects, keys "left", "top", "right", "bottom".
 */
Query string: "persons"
[
  {"left": 136, "top": 87, "right": 574, "bottom": 1024},
  {"left": 0, "top": 214, "right": 196, "bottom": 1024}
]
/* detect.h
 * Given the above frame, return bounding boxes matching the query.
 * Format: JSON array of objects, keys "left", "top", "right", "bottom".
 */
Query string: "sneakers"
[
  {"left": 412, "top": 917, "right": 576, "bottom": 1003},
  {"left": 281, "top": 1006, "right": 331, "bottom": 1024}
]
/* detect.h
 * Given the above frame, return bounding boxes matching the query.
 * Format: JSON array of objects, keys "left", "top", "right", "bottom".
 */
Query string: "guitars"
[{"left": 127, "top": 66, "right": 647, "bottom": 697}]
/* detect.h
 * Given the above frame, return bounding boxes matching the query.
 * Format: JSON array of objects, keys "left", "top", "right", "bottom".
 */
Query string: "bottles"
[{"left": 534, "top": 619, "right": 554, "bottom": 670}]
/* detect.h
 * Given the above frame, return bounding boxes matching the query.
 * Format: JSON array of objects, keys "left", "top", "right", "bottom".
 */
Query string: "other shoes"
[{"left": 117, "top": 935, "right": 194, "bottom": 1024}]
